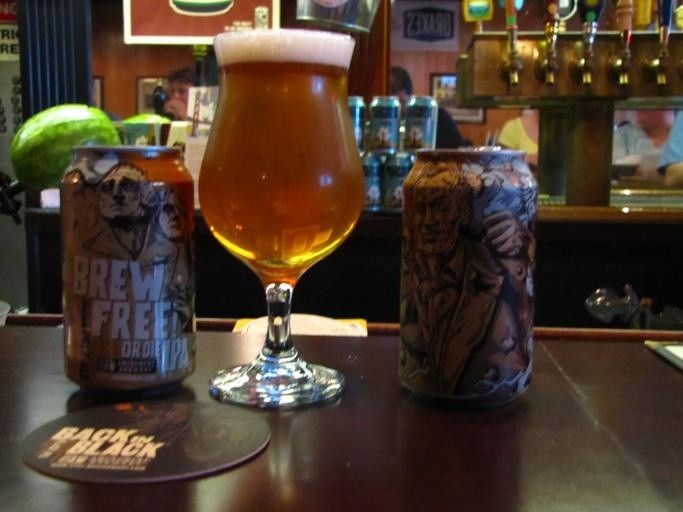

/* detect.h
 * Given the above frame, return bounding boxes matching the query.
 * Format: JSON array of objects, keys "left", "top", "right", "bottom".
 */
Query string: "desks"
[{"left": 0, "top": 311, "right": 681, "bottom": 511}]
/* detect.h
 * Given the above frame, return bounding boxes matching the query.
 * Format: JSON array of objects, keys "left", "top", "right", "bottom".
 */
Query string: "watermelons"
[{"left": 10, "top": 103, "right": 123, "bottom": 191}]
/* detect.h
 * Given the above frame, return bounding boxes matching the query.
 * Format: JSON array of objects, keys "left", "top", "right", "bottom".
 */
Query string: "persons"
[
  {"left": 163, "top": 65, "right": 196, "bottom": 121},
  {"left": 498, "top": 107, "right": 538, "bottom": 168},
  {"left": 390, "top": 64, "right": 462, "bottom": 148},
  {"left": 655, "top": 110, "right": 683, "bottom": 189},
  {"left": 612, "top": 120, "right": 655, "bottom": 167}
]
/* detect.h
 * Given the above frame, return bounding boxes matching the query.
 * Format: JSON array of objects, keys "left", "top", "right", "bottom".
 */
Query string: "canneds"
[
  {"left": 383, "top": 154, "right": 413, "bottom": 212},
  {"left": 402, "top": 94, "right": 438, "bottom": 153},
  {"left": 345, "top": 96, "right": 365, "bottom": 149},
  {"left": 59, "top": 146, "right": 197, "bottom": 390},
  {"left": 399, "top": 147, "right": 539, "bottom": 408},
  {"left": 360, "top": 154, "right": 383, "bottom": 212},
  {"left": 369, "top": 95, "right": 401, "bottom": 154}
]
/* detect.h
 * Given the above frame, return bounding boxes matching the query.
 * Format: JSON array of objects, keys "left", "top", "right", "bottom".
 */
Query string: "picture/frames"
[
  {"left": 134, "top": 75, "right": 171, "bottom": 116},
  {"left": 428, "top": 72, "right": 486, "bottom": 125}
]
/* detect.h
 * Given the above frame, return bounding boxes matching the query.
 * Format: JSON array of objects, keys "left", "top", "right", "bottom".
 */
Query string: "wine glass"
[{"left": 198, "top": 28, "right": 365, "bottom": 409}]
